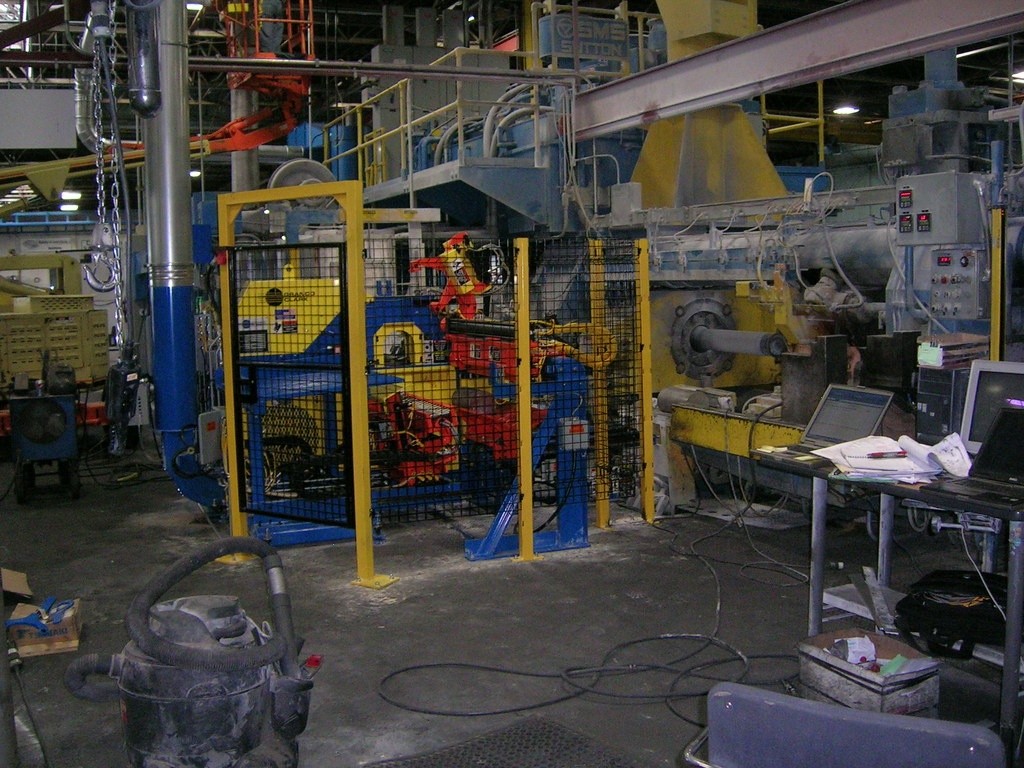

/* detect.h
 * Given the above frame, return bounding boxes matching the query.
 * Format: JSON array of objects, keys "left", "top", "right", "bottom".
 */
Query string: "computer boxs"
[{"left": 918, "top": 360, "right": 972, "bottom": 446}]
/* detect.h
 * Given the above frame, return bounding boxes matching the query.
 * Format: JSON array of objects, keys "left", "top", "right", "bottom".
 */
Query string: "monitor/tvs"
[{"left": 960, "top": 359, "right": 1024, "bottom": 453}]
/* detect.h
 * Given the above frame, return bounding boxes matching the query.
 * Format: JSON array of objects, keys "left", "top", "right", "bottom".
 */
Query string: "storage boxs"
[
  {"left": 10, "top": 598, "right": 83, "bottom": 657},
  {"left": 796, "top": 629, "right": 941, "bottom": 722}
]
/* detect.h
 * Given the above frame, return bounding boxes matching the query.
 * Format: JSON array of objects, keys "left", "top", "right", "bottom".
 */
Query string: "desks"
[{"left": 750, "top": 445, "right": 1024, "bottom": 755}]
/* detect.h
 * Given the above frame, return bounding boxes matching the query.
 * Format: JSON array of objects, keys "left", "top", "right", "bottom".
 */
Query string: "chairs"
[{"left": 683, "top": 682, "right": 1008, "bottom": 768}]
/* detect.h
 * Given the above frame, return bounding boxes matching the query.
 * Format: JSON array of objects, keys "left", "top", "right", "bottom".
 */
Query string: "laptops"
[
  {"left": 752, "top": 384, "right": 895, "bottom": 468},
  {"left": 919, "top": 405, "right": 1024, "bottom": 510}
]
